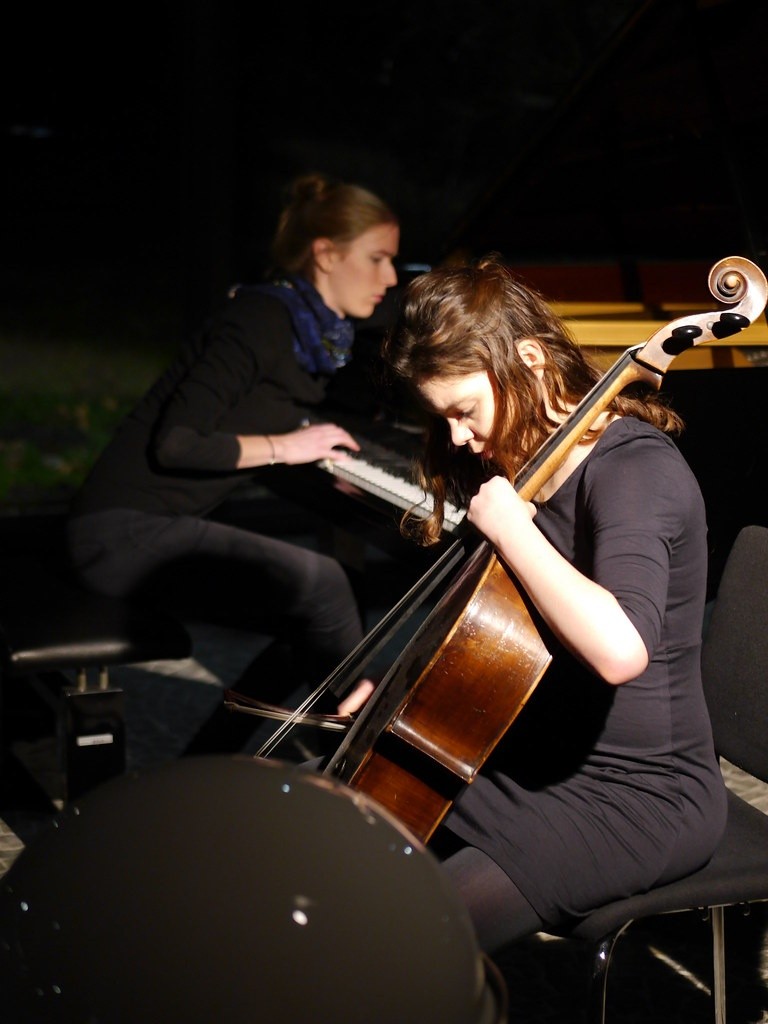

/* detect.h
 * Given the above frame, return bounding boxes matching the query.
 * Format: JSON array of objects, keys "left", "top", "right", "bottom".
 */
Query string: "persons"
[
  {"left": 296, "top": 246, "right": 728, "bottom": 951},
  {"left": 0, "top": 756, "right": 513, "bottom": 1024},
  {"left": 66, "top": 172, "right": 401, "bottom": 681}
]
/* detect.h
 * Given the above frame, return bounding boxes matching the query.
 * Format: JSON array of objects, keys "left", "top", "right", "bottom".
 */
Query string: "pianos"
[{"left": 249, "top": 326, "right": 768, "bottom": 634}]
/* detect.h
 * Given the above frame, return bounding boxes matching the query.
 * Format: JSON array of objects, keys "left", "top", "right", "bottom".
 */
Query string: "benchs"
[{"left": 0, "top": 517, "right": 144, "bottom": 817}]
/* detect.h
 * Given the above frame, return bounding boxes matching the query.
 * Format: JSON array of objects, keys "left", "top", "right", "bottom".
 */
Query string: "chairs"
[
  {"left": 0, "top": 750, "right": 512, "bottom": 1024},
  {"left": 533, "top": 525, "right": 768, "bottom": 1024}
]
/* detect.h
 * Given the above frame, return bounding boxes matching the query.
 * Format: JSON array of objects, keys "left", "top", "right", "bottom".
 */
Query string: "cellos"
[{"left": 252, "top": 256, "right": 768, "bottom": 848}]
